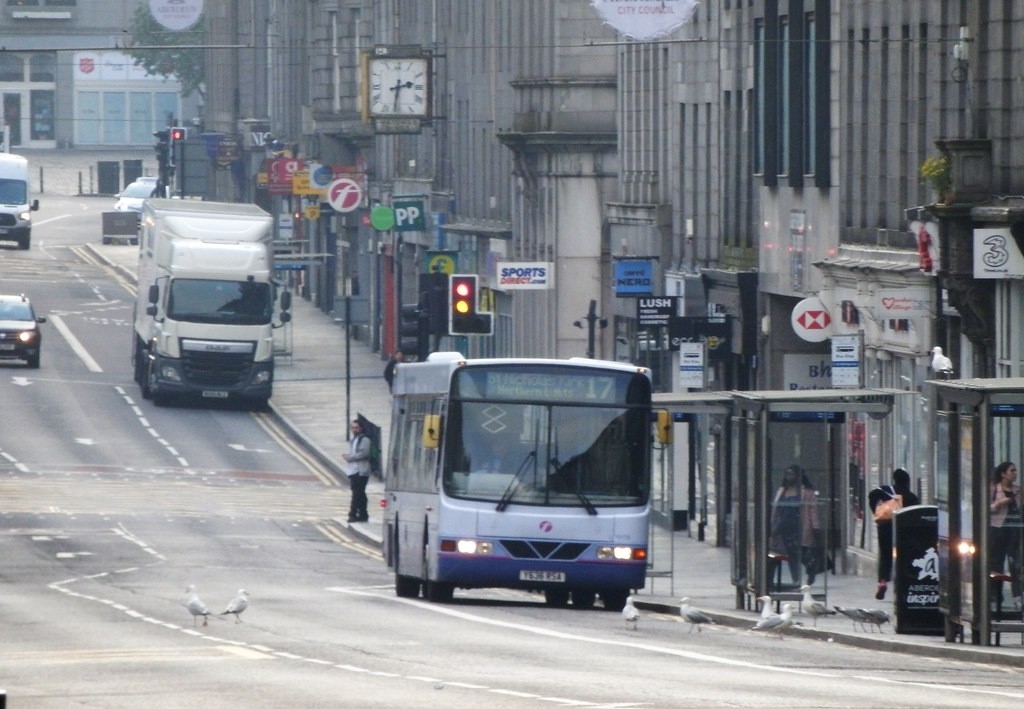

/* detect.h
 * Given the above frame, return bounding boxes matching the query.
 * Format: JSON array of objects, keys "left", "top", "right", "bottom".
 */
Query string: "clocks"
[{"left": 369, "top": 55, "right": 426, "bottom": 116}]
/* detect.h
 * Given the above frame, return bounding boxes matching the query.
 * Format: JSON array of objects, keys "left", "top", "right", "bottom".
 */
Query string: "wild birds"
[
  {"left": 833, "top": 606, "right": 868, "bottom": 633},
  {"left": 220, "top": 589, "right": 250, "bottom": 625},
  {"left": 622, "top": 596, "right": 640, "bottom": 632},
  {"left": 749, "top": 604, "right": 794, "bottom": 639},
  {"left": 679, "top": 597, "right": 715, "bottom": 634},
  {"left": 756, "top": 596, "right": 784, "bottom": 640},
  {"left": 799, "top": 585, "right": 836, "bottom": 627},
  {"left": 858, "top": 608, "right": 890, "bottom": 634},
  {"left": 185, "top": 584, "right": 212, "bottom": 628}
]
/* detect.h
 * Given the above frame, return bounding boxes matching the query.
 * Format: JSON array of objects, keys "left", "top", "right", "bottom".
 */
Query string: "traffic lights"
[
  {"left": 169, "top": 127, "right": 186, "bottom": 167},
  {"left": 448, "top": 275, "right": 494, "bottom": 336},
  {"left": 153, "top": 129, "right": 169, "bottom": 165},
  {"left": 397, "top": 294, "right": 424, "bottom": 356}
]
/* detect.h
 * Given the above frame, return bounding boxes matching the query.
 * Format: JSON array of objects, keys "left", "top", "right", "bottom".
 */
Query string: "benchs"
[
  {"left": 958, "top": 571, "right": 1013, "bottom": 648},
  {"left": 768, "top": 552, "right": 793, "bottom": 614}
]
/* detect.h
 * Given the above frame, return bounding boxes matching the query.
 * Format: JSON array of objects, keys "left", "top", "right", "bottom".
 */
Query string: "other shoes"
[
  {"left": 347, "top": 516, "right": 357, "bottom": 522},
  {"left": 875, "top": 581, "right": 887, "bottom": 600},
  {"left": 357, "top": 516, "right": 367, "bottom": 521},
  {"left": 807, "top": 573, "right": 815, "bottom": 585},
  {"left": 1014, "top": 596, "right": 1023, "bottom": 609}
]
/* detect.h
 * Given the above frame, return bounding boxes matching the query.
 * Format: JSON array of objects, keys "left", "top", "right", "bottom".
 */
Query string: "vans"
[{"left": 0, "top": 152, "right": 39, "bottom": 250}]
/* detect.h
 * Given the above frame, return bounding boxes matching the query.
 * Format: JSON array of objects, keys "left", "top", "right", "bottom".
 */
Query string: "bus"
[{"left": 381, "top": 349, "right": 674, "bottom": 613}]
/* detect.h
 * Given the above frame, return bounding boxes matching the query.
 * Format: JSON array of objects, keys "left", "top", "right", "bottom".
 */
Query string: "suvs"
[{"left": 0, "top": 293, "right": 47, "bottom": 368}]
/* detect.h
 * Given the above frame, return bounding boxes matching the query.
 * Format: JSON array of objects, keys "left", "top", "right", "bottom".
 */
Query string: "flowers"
[{"left": 921, "top": 154, "right": 953, "bottom": 190}]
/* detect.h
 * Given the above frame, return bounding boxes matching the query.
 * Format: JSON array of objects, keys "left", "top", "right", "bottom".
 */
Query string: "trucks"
[
  {"left": 131, "top": 197, "right": 293, "bottom": 410},
  {"left": 112, "top": 176, "right": 165, "bottom": 230}
]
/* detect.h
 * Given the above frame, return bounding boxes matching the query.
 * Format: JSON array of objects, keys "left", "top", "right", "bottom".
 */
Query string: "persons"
[
  {"left": 773, "top": 466, "right": 819, "bottom": 592},
  {"left": 869, "top": 471, "right": 918, "bottom": 599},
  {"left": 991, "top": 462, "right": 1024, "bottom": 607},
  {"left": 384, "top": 350, "right": 405, "bottom": 393},
  {"left": 343, "top": 421, "right": 372, "bottom": 522}
]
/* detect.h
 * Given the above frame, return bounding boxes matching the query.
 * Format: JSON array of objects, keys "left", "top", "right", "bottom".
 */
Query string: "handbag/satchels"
[
  {"left": 874, "top": 487, "right": 902, "bottom": 521},
  {"left": 804, "top": 536, "right": 832, "bottom": 574}
]
